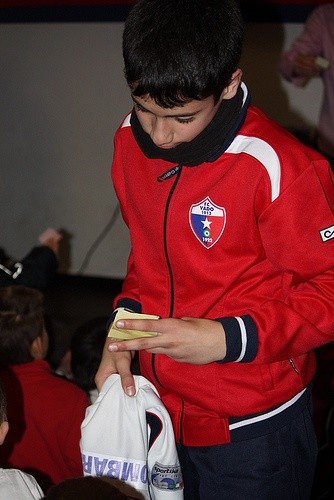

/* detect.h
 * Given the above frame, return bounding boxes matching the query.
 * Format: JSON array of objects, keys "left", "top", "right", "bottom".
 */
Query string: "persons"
[
  {"left": 94, "top": 0, "right": 334, "bottom": 500},
  {"left": 0, "top": 227, "right": 145, "bottom": 499},
  {"left": 281, "top": 0, "right": 334, "bottom": 166}
]
